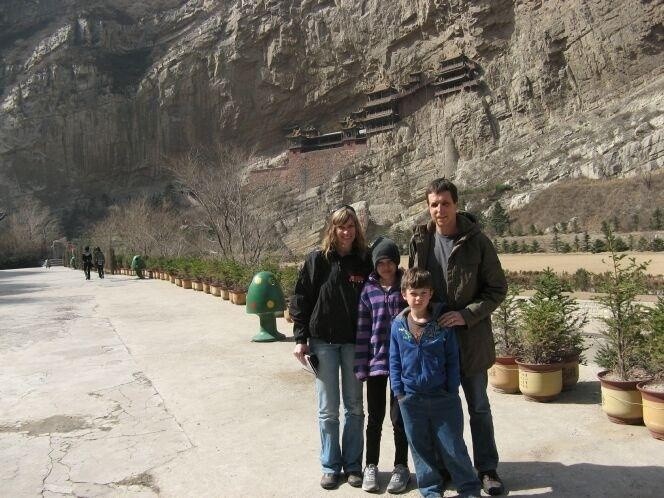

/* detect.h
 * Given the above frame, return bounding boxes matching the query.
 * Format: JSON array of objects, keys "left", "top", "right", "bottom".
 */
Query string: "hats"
[{"left": 371, "top": 237, "right": 400, "bottom": 272}]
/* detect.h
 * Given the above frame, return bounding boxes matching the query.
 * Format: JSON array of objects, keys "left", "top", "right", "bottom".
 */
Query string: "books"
[{"left": 295, "top": 355, "right": 318, "bottom": 376}]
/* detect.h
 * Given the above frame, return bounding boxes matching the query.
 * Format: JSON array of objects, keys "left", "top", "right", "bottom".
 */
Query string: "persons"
[
  {"left": 408, "top": 178, "right": 508, "bottom": 496},
  {"left": 81, "top": 245, "right": 91, "bottom": 280},
  {"left": 387, "top": 267, "right": 485, "bottom": 497},
  {"left": 288, "top": 203, "right": 372, "bottom": 490},
  {"left": 92, "top": 246, "right": 105, "bottom": 279},
  {"left": 352, "top": 236, "right": 412, "bottom": 495}
]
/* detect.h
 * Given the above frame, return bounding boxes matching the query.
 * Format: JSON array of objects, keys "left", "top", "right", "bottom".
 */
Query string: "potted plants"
[
  {"left": 597, "top": 231, "right": 664, "bottom": 440},
  {"left": 488, "top": 269, "right": 582, "bottom": 402},
  {"left": 105, "top": 253, "right": 168, "bottom": 281},
  {"left": 169, "top": 256, "right": 297, "bottom": 323}
]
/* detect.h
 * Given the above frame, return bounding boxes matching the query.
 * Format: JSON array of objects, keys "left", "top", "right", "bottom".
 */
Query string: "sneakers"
[
  {"left": 321, "top": 464, "right": 379, "bottom": 493},
  {"left": 387, "top": 464, "right": 411, "bottom": 493},
  {"left": 478, "top": 470, "right": 504, "bottom": 496}
]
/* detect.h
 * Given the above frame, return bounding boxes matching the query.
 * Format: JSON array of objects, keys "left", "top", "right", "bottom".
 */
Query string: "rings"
[{"left": 449, "top": 319, "right": 453, "bottom": 324}]
